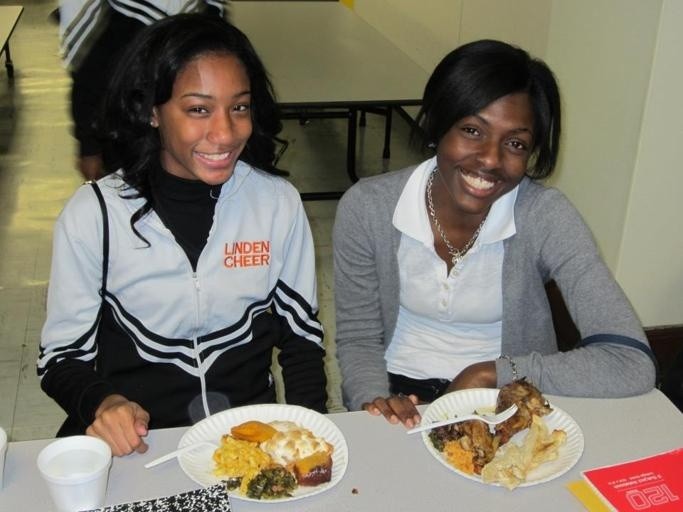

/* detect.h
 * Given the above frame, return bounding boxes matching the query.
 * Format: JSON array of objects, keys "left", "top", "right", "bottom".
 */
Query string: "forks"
[{"left": 407, "top": 403, "right": 519, "bottom": 436}]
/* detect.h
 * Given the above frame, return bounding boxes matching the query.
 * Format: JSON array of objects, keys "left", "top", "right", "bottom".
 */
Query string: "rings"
[{"left": 395, "top": 390, "right": 407, "bottom": 401}]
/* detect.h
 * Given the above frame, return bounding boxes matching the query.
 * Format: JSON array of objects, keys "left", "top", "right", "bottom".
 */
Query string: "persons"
[
  {"left": 328, "top": 35, "right": 662, "bottom": 431},
  {"left": 55, "top": 1, "right": 231, "bottom": 183},
  {"left": 33, "top": 12, "right": 329, "bottom": 461}
]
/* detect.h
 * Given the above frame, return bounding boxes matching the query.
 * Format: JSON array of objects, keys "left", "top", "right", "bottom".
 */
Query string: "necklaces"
[{"left": 426, "top": 167, "right": 492, "bottom": 267}]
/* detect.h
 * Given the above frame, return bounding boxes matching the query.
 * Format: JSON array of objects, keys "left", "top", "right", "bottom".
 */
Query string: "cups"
[{"left": 36, "top": 435, "right": 113, "bottom": 512}]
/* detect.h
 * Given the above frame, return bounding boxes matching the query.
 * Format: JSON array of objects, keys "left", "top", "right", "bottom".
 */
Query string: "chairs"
[{"left": 546, "top": 279, "right": 572, "bottom": 352}]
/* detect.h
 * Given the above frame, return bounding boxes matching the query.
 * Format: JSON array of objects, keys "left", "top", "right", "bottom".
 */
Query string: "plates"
[
  {"left": 421, "top": 387, "right": 584, "bottom": 489},
  {"left": 177, "top": 403, "right": 349, "bottom": 504}
]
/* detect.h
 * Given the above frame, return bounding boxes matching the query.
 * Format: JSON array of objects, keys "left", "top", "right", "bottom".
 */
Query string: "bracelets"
[{"left": 497, "top": 353, "right": 522, "bottom": 385}]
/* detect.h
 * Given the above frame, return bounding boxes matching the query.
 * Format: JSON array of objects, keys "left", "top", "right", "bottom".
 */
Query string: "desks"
[
  {"left": 225, "top": 0, "right": 431, "bottom": 202},
  {"left": 0, "top": 387, "right": 682, "bottom": 512},
  {"left": 1, "top": 6, "right": 24, "bottom": 79}
]
